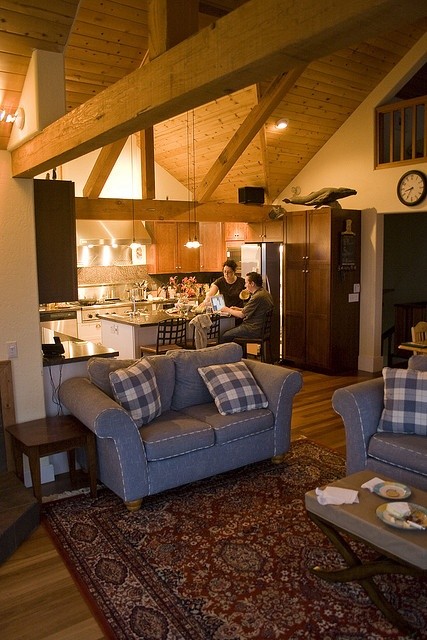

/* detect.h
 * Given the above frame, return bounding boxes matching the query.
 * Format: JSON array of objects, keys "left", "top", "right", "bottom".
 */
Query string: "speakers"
[{"left": 238, "top": 186, "right": 265, "bottom": 205}]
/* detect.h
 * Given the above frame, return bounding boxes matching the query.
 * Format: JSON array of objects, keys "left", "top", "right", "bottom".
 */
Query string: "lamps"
[
  {"left": 130, "top": 237, "right": 141, "bottom": 253},
  {"left": 0, "top": 108, "right": 25, "bottom": 129}
]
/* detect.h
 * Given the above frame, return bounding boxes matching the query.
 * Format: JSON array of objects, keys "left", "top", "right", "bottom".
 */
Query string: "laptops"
[{"left": 210, "top": 295, "right": 232, "bottom": 316}]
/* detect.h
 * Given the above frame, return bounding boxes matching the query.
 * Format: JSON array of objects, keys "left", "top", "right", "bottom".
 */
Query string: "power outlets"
[{"left": 5, "top": 340, "right": 18, "bottom": 359}]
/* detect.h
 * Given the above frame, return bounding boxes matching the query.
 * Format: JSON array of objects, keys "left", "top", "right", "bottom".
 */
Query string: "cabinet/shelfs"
[
  {"left": 200, "top": 221, "right": 227, "bottom": 273},
  {"left": 284, "top": 208, "right": 362, "bottom": 375},
  {"left": 33, "top": 178, "right": 81, "bottom": 304},
  {"left": 244, "top": 219, "right": 284, "bottom": 243},
  {"left": 223, "top": 222, "right": 246, "bottom": 241},
  {"left": 145, "top": 220, "right": 201, "bottom": 275}
]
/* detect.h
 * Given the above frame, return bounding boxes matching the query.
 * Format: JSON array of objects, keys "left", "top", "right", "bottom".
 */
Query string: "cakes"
[{"left": 387, "top": 500, "right": 411, "bottom": 518}]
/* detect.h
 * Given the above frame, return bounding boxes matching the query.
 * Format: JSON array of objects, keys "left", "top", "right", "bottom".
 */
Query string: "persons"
[
  {"left": 199, "top": 260, "right": 252, "bottom": 327},
  {"left": 220, "top": 272, "right": 274, "bottom": 344}
]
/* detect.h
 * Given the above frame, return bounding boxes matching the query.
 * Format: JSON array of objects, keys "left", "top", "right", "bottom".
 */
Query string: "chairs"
[
  {"left": 138, "top": 317, "right": 187, "bottom": 357},
  {"left": 411, "top": 322, "right": 426, "bottom": 356},
  {"left": 233, "top": 309, "right": 274, "bottom": 365},
  {"left": 176, "top": 313, "right": 220, "bottom": 349}
]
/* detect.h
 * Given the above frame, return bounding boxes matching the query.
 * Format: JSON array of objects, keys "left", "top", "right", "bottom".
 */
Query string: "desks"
[{"left": 5, "top": 413, "right": 98, "bottom": 505}]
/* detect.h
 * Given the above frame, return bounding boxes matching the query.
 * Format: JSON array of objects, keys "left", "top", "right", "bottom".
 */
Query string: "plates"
[
  {"left": 373, "top": 481, "right": 412, "bottom": 500},
  {"left": 375, "top": 501, "right": 427, "bottom": 530}
]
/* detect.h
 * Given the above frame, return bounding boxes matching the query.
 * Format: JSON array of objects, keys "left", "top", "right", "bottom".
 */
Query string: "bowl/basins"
[{"left": 78, "top": 299, "right": 97, "bottom": 304}]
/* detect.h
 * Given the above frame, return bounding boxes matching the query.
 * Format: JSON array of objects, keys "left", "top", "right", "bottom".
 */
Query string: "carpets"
[{"left": 40, "top": 439, "right": 426, "bottom": 638}]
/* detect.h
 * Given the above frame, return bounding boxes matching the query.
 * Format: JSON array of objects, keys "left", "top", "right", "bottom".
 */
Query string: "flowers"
[{"left": 167, "top": 275, "right": 203, "bottom": 315}]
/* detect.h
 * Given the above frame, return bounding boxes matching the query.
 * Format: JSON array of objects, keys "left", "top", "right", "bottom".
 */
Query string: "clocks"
[{"left": 397, "top": 170, "right": 427, "bottom": 206}]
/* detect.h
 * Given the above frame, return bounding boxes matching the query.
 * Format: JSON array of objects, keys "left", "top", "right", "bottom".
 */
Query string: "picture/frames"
[{"left": 338, "top": 231, "right": 357, "bottom": 272}]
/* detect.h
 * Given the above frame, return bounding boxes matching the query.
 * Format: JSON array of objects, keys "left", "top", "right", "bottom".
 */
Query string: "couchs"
[
  {"left": 332, "top": 354, "right": 427, "bottom": 491},
  {"left": 54, "top": 342, "right": 303, "bottom": 511}
]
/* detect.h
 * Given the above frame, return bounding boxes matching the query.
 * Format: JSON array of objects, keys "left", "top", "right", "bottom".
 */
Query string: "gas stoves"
[{"left": 70, "top": 302, "right": 133, "bottom": 311}]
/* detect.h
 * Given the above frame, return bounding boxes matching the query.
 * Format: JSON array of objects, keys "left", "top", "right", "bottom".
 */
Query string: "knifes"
[{"left": 388, "top": 513, "right": 426, "bottom": 530}]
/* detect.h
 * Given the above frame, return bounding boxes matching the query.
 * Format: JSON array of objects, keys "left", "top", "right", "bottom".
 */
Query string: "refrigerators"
[{"left": 240, "top": 243, "right": 282, "bottom": 362}]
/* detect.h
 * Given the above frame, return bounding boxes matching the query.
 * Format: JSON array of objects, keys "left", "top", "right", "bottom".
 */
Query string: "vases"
[{"left": 174, "top": 304, "right": 190, "bottom": 320}]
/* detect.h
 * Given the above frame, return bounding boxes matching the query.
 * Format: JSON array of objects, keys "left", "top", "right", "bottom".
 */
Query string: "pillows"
[
  {"left": 197, "top": 360, "right": 269, "bottom": 416},
  {"left": 376, "top": 365, "right": 427, "bottom": 438},
  {"left": 108, "top": 356, "right": 163, "bottom": 429}
]
